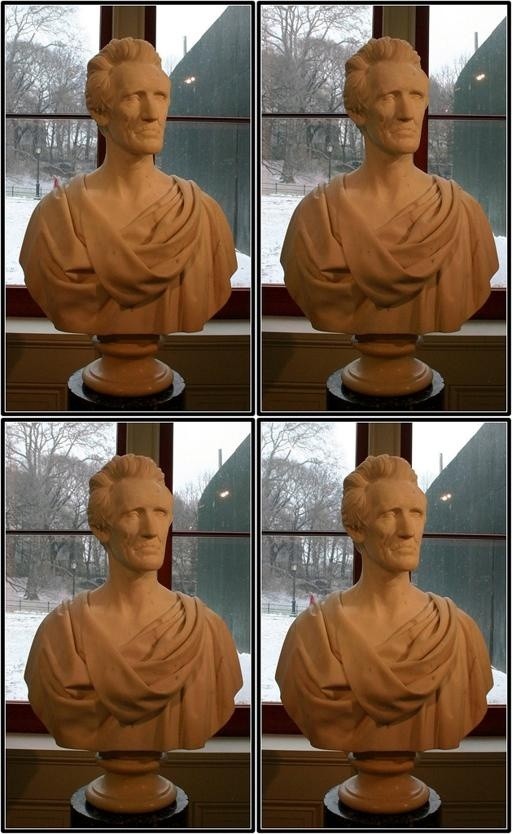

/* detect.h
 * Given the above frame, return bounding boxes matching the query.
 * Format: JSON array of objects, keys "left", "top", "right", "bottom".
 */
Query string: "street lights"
[
  {"left": 35, "top": 146, "right": 40, "bottom": 200},
  {"left": 326, "top": 143, "right": 333, "bottom": 183},
  {"left": 290, "top": 563, "right": 299, "bottom": 614},
  {"left": 70, "top": 560, "right": 77, "bottom": 597}
]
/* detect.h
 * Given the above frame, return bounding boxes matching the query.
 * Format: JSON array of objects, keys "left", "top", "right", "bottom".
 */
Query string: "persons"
[
  {"left": 18, "top": 36, "right": 238, "bottom": 336},
  {"left": 274, "top": 453, "right": 494, "bottom": 753},
  {"left": 279, "top": 35, "right": 500, "bottom": 337},
  {"left": 23, "top": 455, "right": 244, "bottom": 752}
]
